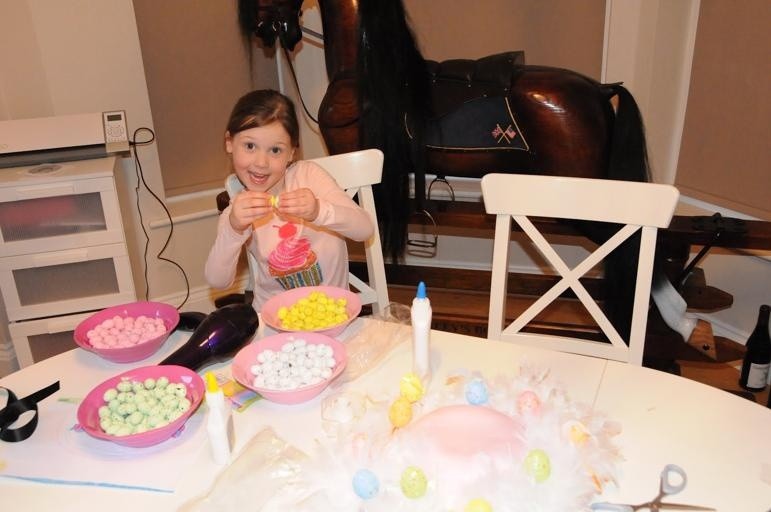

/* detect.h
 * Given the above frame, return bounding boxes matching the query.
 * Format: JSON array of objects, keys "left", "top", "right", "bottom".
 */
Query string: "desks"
[{"left": 0, "top": 313, "right": 771, "bottom": 512}]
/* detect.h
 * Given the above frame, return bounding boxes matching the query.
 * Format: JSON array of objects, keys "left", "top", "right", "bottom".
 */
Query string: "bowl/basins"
[
  {"left": 76, "top": 365, "right": 206, "bottom": 448},
  {"left": 261, "top": 285, "right": 362, "bottom": 338},
  {"left": 73, "top": 301, "right": 181, "bottom": 364},
  {"left": 231, "top": 332, "right": 348, "bottom": 405}
]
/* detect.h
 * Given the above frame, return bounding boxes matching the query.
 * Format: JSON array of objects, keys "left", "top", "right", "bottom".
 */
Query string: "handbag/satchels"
[{"left": 405, "top": 52, "right": 534, "bottom": 151}]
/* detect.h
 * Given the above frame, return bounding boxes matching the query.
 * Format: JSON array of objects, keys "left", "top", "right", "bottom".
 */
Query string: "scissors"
[{"left": 589, "top": 463, "right": 717, "bottom": 511}]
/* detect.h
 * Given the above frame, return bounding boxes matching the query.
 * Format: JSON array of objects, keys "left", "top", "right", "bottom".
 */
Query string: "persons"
[{"left": 204, "top": 89, "right": 375, "bottom": 313}]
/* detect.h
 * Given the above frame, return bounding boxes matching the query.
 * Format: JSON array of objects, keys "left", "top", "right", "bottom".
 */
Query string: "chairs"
[
  {"left": 226, "top": 150, "right": 391, "bottom": 316},
  {"left": 481, "top": 173, "right": 679, "bottom": 367}
]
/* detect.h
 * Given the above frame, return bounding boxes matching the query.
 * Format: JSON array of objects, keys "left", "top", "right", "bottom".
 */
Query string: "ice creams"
[{"left": 268, "top": 223, "right": 323, "bottom": 289}]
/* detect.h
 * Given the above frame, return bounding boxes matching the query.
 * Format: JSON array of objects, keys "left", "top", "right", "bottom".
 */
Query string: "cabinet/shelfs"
[{"left": 0, "top": 157, "right": 139, "bottom": 372}]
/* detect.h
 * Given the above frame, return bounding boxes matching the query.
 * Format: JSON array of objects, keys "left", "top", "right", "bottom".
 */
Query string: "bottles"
[
  {"left": 741, "top": 304, "right": 771, "bottom": 392},
  {"left": 205, "top": 371, "right": 237, "bottom": 465},
  {"left": 410, "top": 281, "right": 434, "bottom": 385}
]
